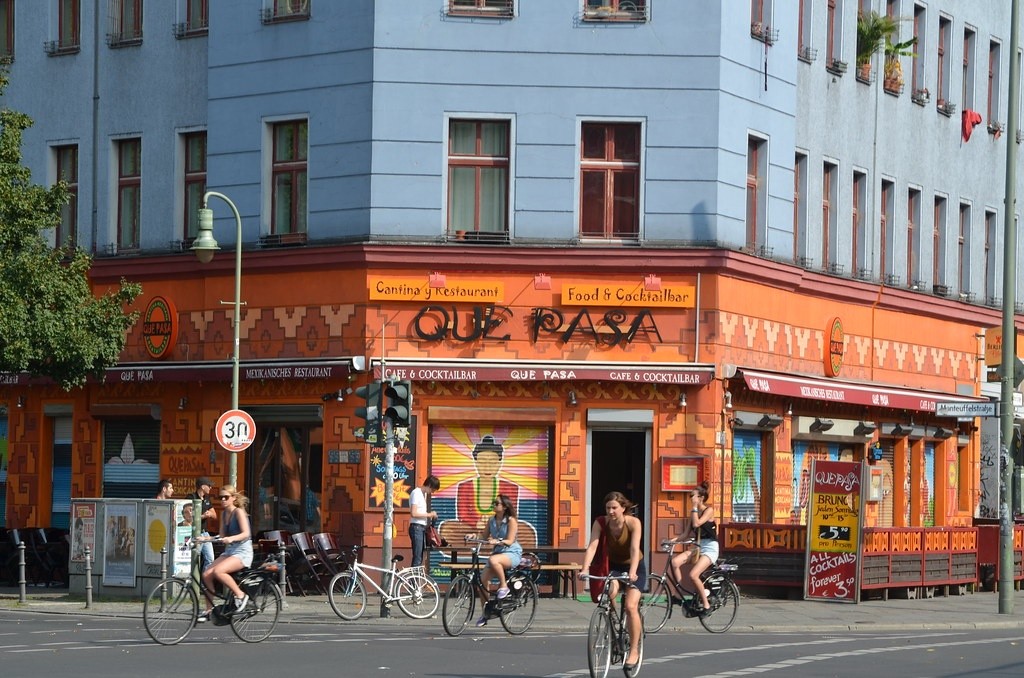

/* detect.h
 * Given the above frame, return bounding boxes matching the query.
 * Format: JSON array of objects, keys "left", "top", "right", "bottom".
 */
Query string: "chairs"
[{"left": 252, "top": 528, "right": 348, "bottom": 596}]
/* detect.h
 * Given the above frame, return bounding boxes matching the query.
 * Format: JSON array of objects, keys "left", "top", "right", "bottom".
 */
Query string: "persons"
[
  {"left": 186, "top": 477, "right": 218, "bottom": 596},
  {"left": 193, "top": 485, "right": 254, "bottom": 622},
  {"left": 154, "top": 479, "right": 174, "bottom": 500},
  {"left": 671, "top": 480, "right": 721, "bottom": 619},
  {"left": 464, "top": 493, "right": 523, "bottom": 628},
  {"left": 6, "top": 526, "right": 69, "bottom": 589},
  {"left": 408, "top": 474, "right": 440, "bottom": 594},
  {"left": 74, "top": 516, "right": 85, "bottom": 554},
  {"left": 579, "top": 490, "right": 648, "bottom": 670},
  {"left": 180, "top": 503, "right": 195, "bottom": 525}
]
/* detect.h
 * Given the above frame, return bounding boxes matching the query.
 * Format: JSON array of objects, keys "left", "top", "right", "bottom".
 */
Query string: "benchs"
[{"left": 440, "top": 562, "right": 583, "bottom": 601}]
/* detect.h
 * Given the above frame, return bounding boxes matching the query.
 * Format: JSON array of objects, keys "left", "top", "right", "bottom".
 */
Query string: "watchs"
[{"left": 691, "top": 508, "right": 698, "bottom": 512}]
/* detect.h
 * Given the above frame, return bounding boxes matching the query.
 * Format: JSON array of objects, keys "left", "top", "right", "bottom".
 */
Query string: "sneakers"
[
  {"left": 233, "top": 593, "right": 249, "bottom": 614},
  {"left": 497, "top": 586, "right": 510, "bottom": 599},
  {"left": 476, "top": 618, "right": 486, "bottom": 627},
  {"left": 196, "top": 611, "right": 211, "bottom": 622}
]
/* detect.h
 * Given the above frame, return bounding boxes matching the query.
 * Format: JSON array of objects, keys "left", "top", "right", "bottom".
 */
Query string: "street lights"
[{"left": 188, "top": 191, "right": 242, "bottom": 491}]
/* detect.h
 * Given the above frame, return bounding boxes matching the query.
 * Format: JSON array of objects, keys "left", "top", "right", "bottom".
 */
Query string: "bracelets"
[{"left": 497, "top": 538, "right": 501, "bottom": 545}]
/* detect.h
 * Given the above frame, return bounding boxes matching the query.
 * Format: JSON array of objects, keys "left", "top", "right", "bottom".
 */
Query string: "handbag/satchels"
[
  {"left": 588, "top": 516, "right": 610, "bottom": 604},
  {"left": 424, "top": 518, "right": 442, "bottom": 549},
  {"left": 690, "top": 548, "right": 700, "bottom": 565}
]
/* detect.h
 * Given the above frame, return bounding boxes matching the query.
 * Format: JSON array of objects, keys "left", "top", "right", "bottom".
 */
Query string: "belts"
[{"left": 201, "top": 529, "right": 209, "bottom": 533}]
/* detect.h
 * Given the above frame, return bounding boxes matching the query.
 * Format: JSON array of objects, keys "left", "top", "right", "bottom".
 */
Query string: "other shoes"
[
  {"left": 664, "top": 596, "right": 682, "bottom": 606},
  {"left": 623, "top": 654, "right": 639, "bottom": 670},
  {"left": 699, "top": 606, "right": 714, "bottom": 619}
]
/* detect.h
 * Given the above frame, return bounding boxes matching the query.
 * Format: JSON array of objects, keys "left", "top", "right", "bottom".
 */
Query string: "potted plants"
[{"left": 852, "top": 8, "right": 919, "bottom": 93}]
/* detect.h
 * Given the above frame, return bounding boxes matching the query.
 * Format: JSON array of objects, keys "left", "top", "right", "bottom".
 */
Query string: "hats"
[{"left": 196, "top": 477, "right": 215, "bottom": 489}]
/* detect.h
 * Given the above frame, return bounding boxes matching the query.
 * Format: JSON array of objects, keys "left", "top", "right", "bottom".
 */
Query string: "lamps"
[
  {"left": 758, "top": 415, "right": 784, "bottom": 427},
  {"left": 933, "top": 428, "right": 953, "bottom": 438},
  {"left": 893, "top": 424, "right": 913, "bottom": 435},
  {"left": 854, "top": 422, "right": 877, "bottom": 437},
  {"left": 336, "top": 389, "right": 344, "bottom": 402},
  {"left": 810, "top": 419, "right": 834, "bottom": 432},
  {"left": 678, "top": 394, "right": 687, "bottom": 407},
  {"left": 178, "top": 397, "right": 185, "bottom": 410},
  {"left": 568, "top": 390, "right": 578, "bottom": 405}
]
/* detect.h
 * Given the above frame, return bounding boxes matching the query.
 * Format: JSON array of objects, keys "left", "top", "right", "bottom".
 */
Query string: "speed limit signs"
[{"left": 215, "top": 409, "right": 257, "bottom": 452}]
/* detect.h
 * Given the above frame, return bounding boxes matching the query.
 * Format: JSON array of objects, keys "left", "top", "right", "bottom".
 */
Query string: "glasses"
[
  {"left": 491, "top": 501, "right": 502, "bottom": 507},
  {"left": 218, "top": 495, "right": 233, "bottom": 501},
  {"left": 690, "top": 495, "right": 701, "bottom": 498}
]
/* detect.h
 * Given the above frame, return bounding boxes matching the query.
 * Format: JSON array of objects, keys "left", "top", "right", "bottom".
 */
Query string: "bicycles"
[
  {"left": 143, "top": 535, "right": 284, "bottom": 646},
  {"left": 442, "top": 533, "right": 539, "bottom": 636},
  {"left": 578, "top": 572, "right": 646, "bottom": 678},
  {"left": 637, "top": 539, "right": 742, "bottom": 632},
  {"left": 328, "top": 545, "right": 440, "bottom": 620}
]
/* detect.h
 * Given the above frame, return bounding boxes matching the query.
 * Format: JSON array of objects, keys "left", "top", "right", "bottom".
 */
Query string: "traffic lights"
[
  {"left": 384, "top": 380, "right": 412, "bottom": 428},
  {"left": 353, "top": 383, "right": 383, "bottom": 445}
]
